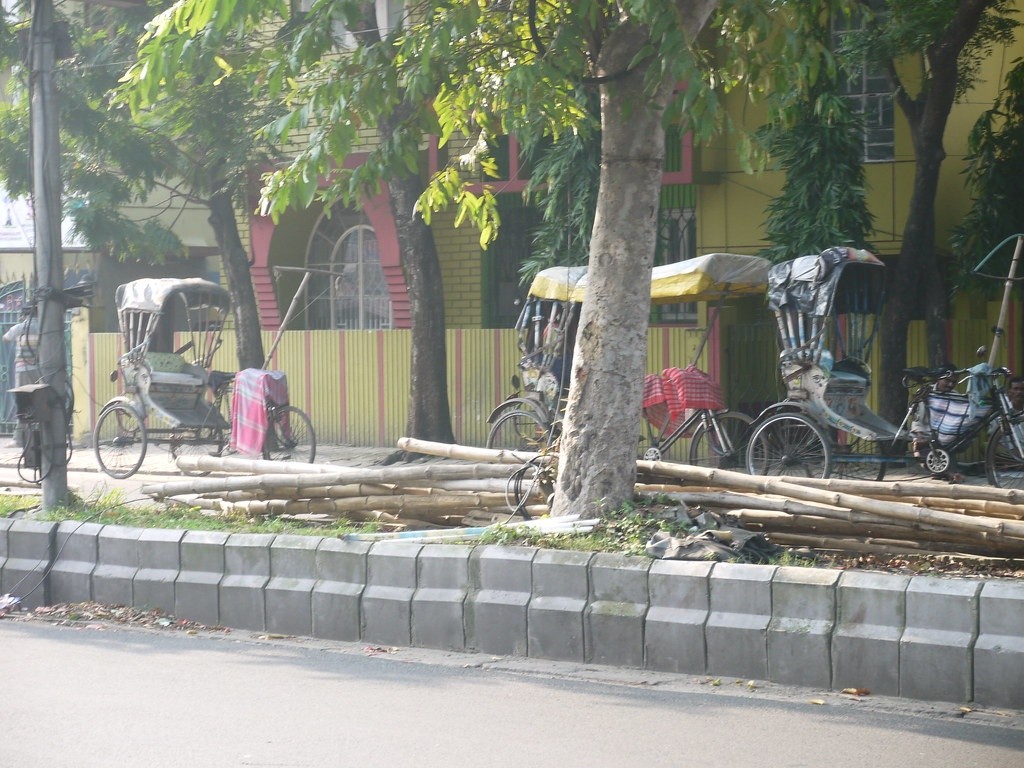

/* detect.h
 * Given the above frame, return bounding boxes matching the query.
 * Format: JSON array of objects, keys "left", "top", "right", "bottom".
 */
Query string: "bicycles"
[{"left": 485, "top": 253, "right": 774, "bottom": 478}]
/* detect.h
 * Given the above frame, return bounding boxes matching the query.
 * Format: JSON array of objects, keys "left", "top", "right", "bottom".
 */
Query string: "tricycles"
[
  {"left": 91, "top": 276, "right": 318, "bottom": 480},
  {"left": 746, "top": 244, "right": 1024, "bottom": 490}
]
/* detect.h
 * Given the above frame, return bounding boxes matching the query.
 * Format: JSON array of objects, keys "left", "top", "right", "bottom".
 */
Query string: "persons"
[
  {"left": 911, "top": 363, "right": 967, "bottom": 484},
  {"left": 2, "top": 300, "right": 41, "bottom": 448},
  {"left": 987, "top": 375, "right": 1024, "bottom": 473}
]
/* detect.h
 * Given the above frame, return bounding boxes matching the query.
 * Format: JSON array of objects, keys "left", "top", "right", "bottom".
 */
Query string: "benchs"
[
  {"left": 129, "top": 351, "right": 203, "bottom": 409},
  {"left": 779, "top": 347, "right": 873, "bottom": 396},
  {"left": 529, "top": 340, "right": 572, "bottom": 408}
]
